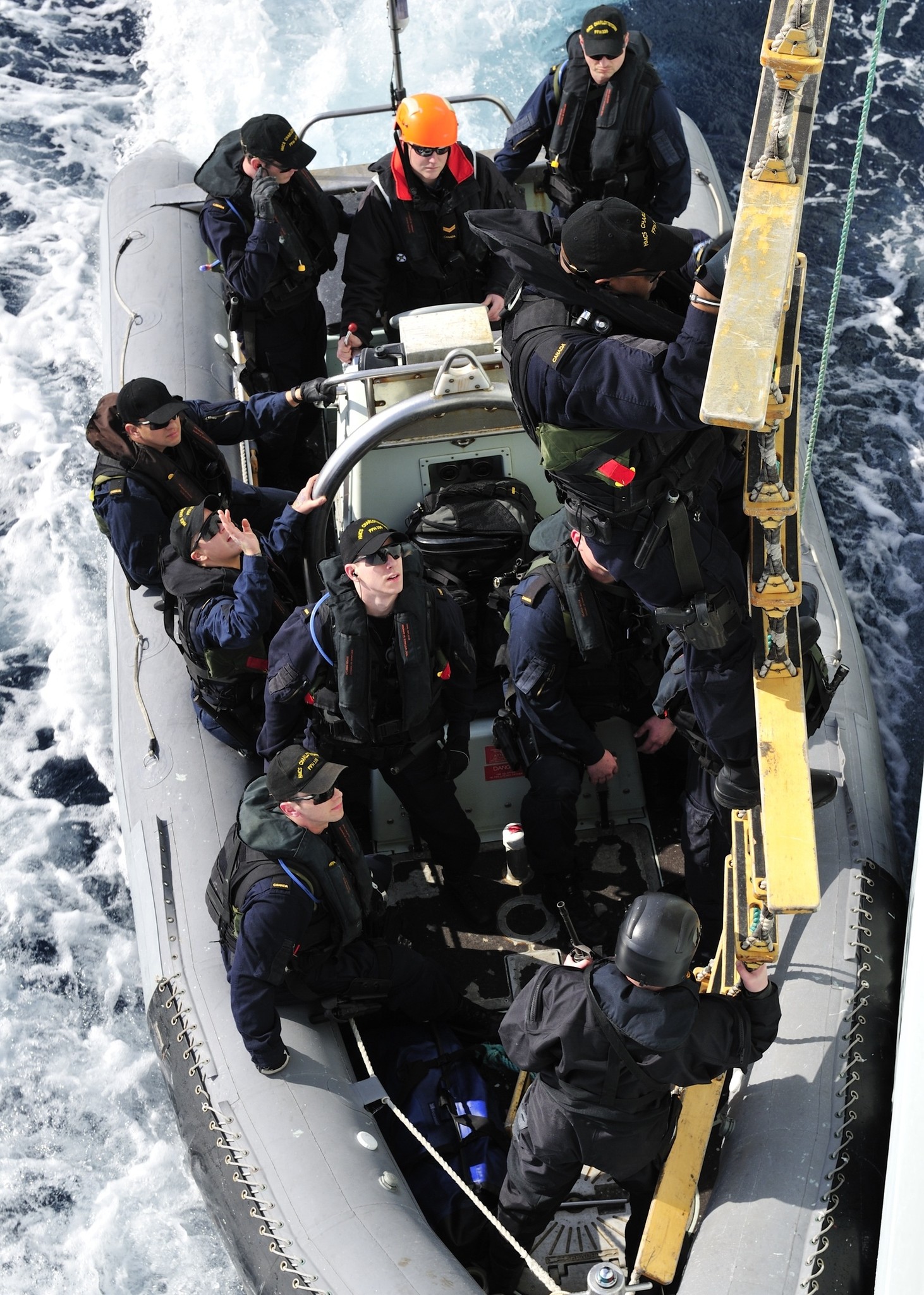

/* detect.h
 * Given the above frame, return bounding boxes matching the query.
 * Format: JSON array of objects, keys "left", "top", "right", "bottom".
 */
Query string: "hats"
[
  {"left": 116, "top": 377, "right": 189, "bottom": 425},
  {"left": 581, "top": 5, "right": 627, "bottom": 56},
  {"left": 266, "top": 744, "right": 349, "bottom": 803},
  {"left": 560, "top": 197, "right": 693, "bottom": 281},
  {"left": 339, "top": 517, "right": 409, "bottom": 565},
  {"left": 170, "top": 494, "right": 221, "bottom": 562},
  {"left": 241, "top": 114, "right": 316, "bottom": 169}
]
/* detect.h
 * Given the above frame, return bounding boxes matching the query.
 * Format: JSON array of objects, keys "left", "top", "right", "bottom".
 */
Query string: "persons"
[
  {"left": 499, "top": 485, "right": 638, "bottom": 946},
  {"left": 153, "top": 474, "right": 328, "bottom": 769},
  {"left": 192, "top": 4, "right": 692, "bottom": 497},
  {"left": 501, "top": 195, "right": 846, "bottom": 809},
  {"left": 633, "top": 625, "right": 829, "bottom": 932},
  {"left": 480, "top": 890, "right": 783, "bottom": 1294},
  {"left": 85, "top": 378, "right": 337, "bottom": 598},
  {"left": 256, "top": 516, "right": 483, "bottom": 948},
  {"left": 201, "top": 744, "right": 452, "bottom": 1078}
]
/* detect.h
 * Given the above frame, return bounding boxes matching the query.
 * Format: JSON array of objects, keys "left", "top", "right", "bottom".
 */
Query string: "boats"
[{"left": 96, "top": 1, "right": 911, "bottom": 1293}]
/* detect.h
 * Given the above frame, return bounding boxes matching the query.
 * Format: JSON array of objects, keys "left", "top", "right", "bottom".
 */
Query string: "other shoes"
[{"left": 487, "top": 1234, "right": 527, "bottom": 1295}]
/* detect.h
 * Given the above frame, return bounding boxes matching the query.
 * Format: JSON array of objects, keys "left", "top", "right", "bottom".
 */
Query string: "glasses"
[
  {"left": 191, "top": 510, "right": 222, "bottom": 553},
  {"left": 352, "top": 542, "right": 404, "bottom": 565},
  {"left": 133, "top": 412, "right": 180, "bottom": 430},
  {"left": 612, "top": 267, "right": 662, "bottom": 284},
  {"left": 406, "top": 142, "right": 450, "bottom": 156},
  {"left": 286, "top": 777, "right": 341, "bottom": 806},
  {"left": 588, "top": 50, "right": 623, "bottom": 61},
  {"left": 251, "top": 154, "right": 293, "bottom": 173}
]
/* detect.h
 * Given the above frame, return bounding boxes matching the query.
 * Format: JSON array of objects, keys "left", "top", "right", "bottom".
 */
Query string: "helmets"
[
  {"left": 394, "top": 94, "right": 458, "bottom": 147},
  {"left": 614, "top": 891, "right": 703, "bottom": 987}
]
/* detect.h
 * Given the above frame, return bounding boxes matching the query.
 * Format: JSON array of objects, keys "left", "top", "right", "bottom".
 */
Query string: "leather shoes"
[
  {"left": 713, "top": 765, "right": 838, "bottom": 810},
  {"left": 438, "top": 864, "right": 721, "bottom": 962},
  {"left": 446, "top": 993, "right": 504, "bottom": 1039}
]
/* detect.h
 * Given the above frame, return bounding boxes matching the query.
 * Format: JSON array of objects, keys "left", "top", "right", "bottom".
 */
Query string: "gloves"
[
  {"left": 255, "top": 1046, "right": 290, "bottom": 1075},
  {"left": 368, "top": 867, "right": 388, "bottom": 922},
  {"left": 435, "top": 736, "right": 470, "bottom": 782},
  {"left": 693, "top": 239, "right": 725, "bottom": 300},
  {"left": 250, "top": 167, "right": 280, "bottom": 224},
  {"left": 300, "top": 376, "right": 337, "bottom": 408},
  {"left": 695, "top": 229, "right": 734, "bottom": 268}
]
